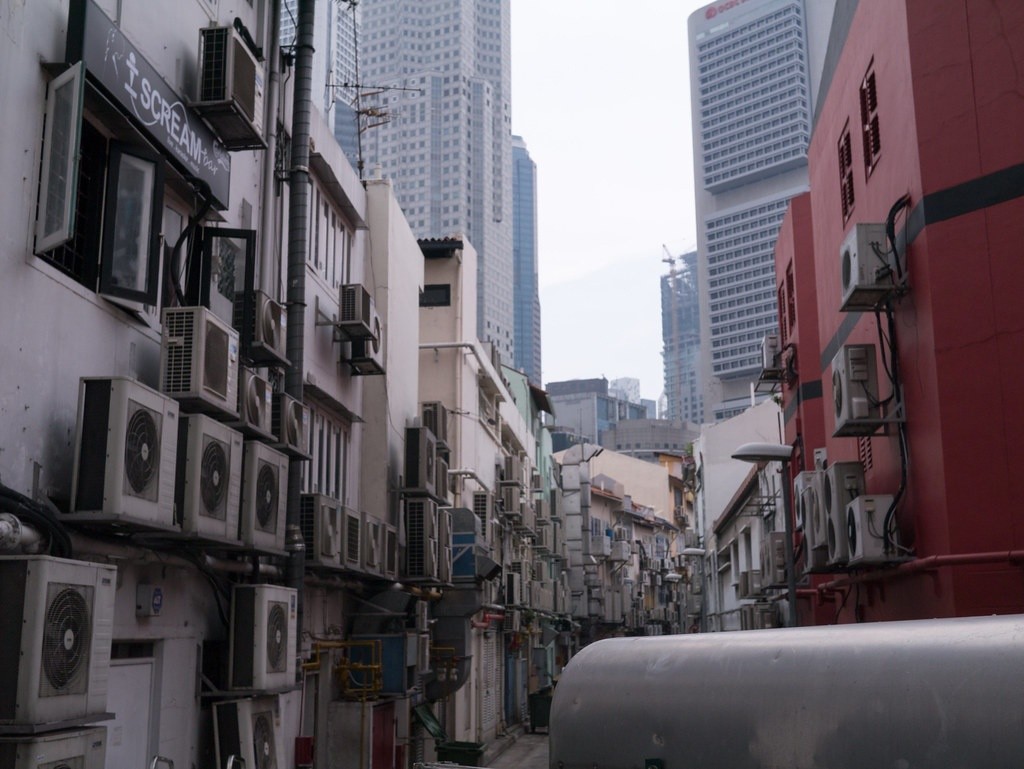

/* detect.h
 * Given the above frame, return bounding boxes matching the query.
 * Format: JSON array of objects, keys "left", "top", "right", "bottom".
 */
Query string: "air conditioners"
[
  {"left": 352, "top": 313, "right": 383, "bottom": 368},
  {"left": 158, "top": 305, "right": 240, "bottom": 414},
  {"left": 793, "top": 459, "right": 898, "bottom": 574},
  {"left": 1, "top": 555, "right": 118, "bottom": 725},
  {"left": 212, "top": 694, "right": 287, "bottom": 769},
  {"left": 339, "top": 283, "right": 376, "bottom": 334},
  {"left": 170, "top": 413, "right": 244, "bottom": 541},
  {"left": 343, "top": 505, "right": 363, "bottom": 572},
  {"left": 286, "top": 493, "right": 342, "bottom": 573},
  {"left": 238, "top": 439, "right": 291, "bottom": 552},
  {"left": 233, "top": 289, "right": 283, "bottom": 353},
  {"left": 831, "top": 343, "right": 881, "bottom": 430},
  {"left": 361, "top": 400, "right": 788, "bottom": 693},
  {"left": 70, "top": 374, "right": 182, "bottom": 528},
  {"left": 838, "top": 222, "right": 890, "bottom": 306},
  {"left": 238, "top": 366, "right": 273, "bottom": 434},
  {"left": 1, "top": 727, "right": 109, "bottom": 769},
  {"left": 260, "top": 392, "right": 311, "bottom": 454},
  {"left": 194, "top": 24, "right": 266, "bottom": 137},
  {"left": 228, "top": 584, "right": 300, "bottom": 691},
  {"left": 760, "top": 334, "right": 783, "bottom": 380}
]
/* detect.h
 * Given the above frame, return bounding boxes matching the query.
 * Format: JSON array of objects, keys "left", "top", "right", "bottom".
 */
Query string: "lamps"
[
  {"left": 677, "top": 548, "right": 704, "bottom": 557},
  {"left": 664, "top": 573, "right": 683, "bottom": 580},
  {"left": 731, "top": 441, "right": 793, "bottom": 462}
]
